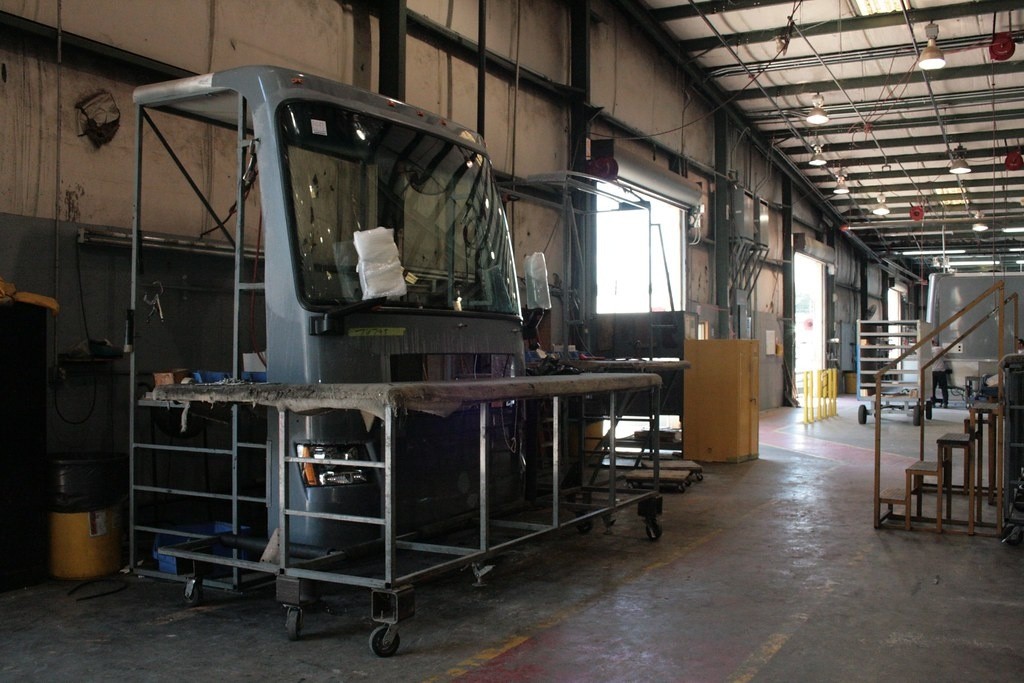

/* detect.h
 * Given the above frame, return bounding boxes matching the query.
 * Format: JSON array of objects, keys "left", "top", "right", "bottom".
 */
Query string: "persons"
[
  {"left": 1018, "top": 339, "right": 1024, "bottom": 354},
  {"left": 928, "top": 338, "right": 950, "bottom": 409}
]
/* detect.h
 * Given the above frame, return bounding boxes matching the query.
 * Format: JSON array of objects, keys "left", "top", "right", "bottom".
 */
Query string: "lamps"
[
  {"left": 806, "top": 92, "right": 829, "bottom": 124},
  {"left": 834, "top": 167, "right": 850, "bottom": 194},
  {"left": 949, "top": 143, "right": 972, "bottom": 174},
  {"left": 918, "top": 21, "right": 945, "bottom": 69},
  {"left": 971, "top": 209, "right": 988, "bottom": 231},
  {"left": 808, "top": 135, "right": 827, "bottom": 165},
  {"left": 873, "top": 192, "right": 890, "bottom": 215}
]
